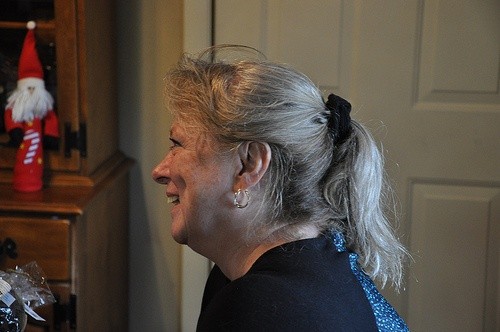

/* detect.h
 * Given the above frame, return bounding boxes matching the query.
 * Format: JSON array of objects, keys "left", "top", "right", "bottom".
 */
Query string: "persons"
[{"left": 151, "top": 43, "right": 420, "bottom": 332}]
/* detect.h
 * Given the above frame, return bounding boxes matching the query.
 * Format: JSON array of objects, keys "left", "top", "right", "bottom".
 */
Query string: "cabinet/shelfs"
[
  {"left": 0, "top": 0, "right": 125, "bottom": 187},
  {"left": 0, "top": 158, "right": 135, "bottom": 332}
]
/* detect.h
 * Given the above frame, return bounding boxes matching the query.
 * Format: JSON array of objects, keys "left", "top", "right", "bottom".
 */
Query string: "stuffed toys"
[{"left": 4, "top": 20, "right": 63, "bottom": 192}]
